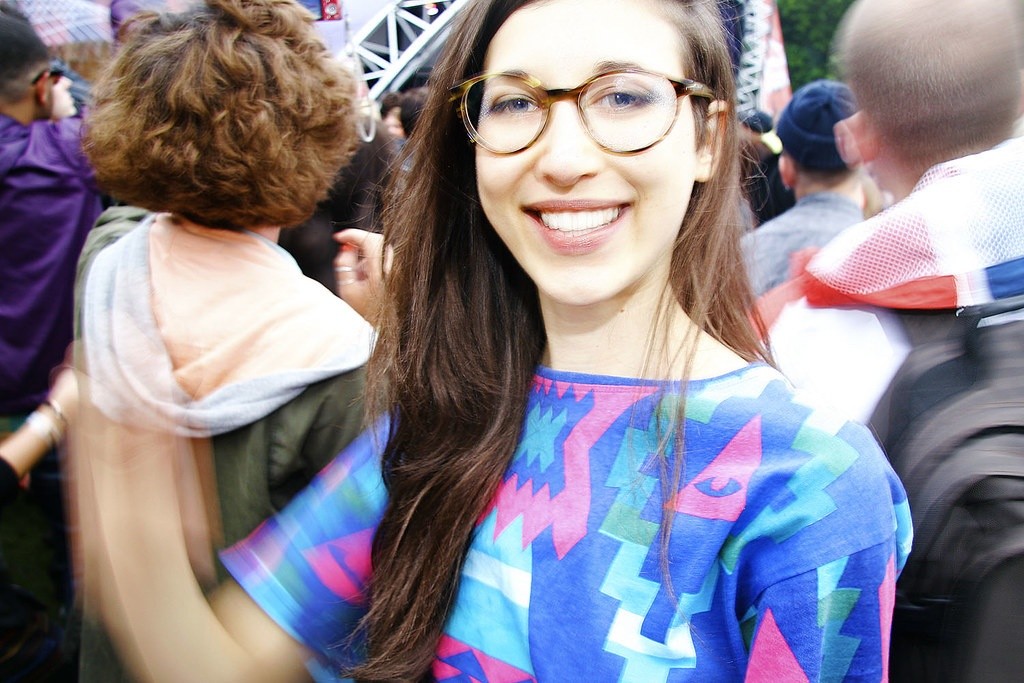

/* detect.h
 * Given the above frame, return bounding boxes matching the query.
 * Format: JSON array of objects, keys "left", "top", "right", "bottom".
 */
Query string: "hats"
[{"left": 777, "top": 79, "right": 858, "bottom": 170}]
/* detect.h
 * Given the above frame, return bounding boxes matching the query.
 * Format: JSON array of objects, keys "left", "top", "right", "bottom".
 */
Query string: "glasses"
[
  {"left": 31, "top": 68, "right": 63, "bottom": 85},
  {"left": 446, "top": 69, "right": 716, "bottom": 156}
]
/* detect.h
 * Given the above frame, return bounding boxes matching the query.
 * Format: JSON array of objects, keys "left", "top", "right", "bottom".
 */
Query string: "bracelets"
[{"left": 24, "top": 397, "right": 75, "bottom": 452}]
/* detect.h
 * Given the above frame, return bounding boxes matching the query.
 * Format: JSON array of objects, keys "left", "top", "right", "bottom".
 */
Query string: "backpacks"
[{"left": 870, "top": 308, "right": 1022, "bottom": 683}]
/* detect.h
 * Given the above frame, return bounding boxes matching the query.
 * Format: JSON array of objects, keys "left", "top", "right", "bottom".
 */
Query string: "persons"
[
  {"left": 748, "top": 5, "right": 1024, "bottom": 683},
  {"left": 45, "top": 0, "right": 403, "bottom": 683},
  {"left": 73, "top": 0, "right": 920, "bottom": 683},
  {"left": 0, "top": 0, "right": 109, "bottom": 581},
  {"left": 1, "top": 366, "right": 84, "bottom": 682},
  {"left": 25, "top": 1, "right": 904, "bottom": 303}
]
